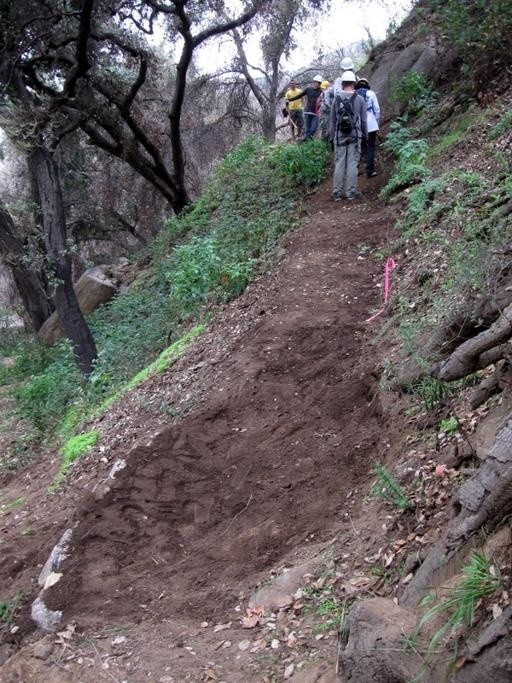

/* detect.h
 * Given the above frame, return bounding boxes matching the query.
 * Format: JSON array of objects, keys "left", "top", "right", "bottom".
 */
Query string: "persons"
[
  {"left": 327, "top": 70, "right": 364, "bottom": 200},
  {"left": 283, "top": 57, "right": 382, "bottom": 176}
]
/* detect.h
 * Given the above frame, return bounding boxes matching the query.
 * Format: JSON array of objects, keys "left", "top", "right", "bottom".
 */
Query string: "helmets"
[
  {"left": 320, "top": 80, "right": 330, "bottom": 90},
  {"left": 313, "top": 74, "right": 324, "bottom": 82},
  {"left": 356, "top": 77, "right": 371, "bottom": 88},
  {"left": 340, "top": 56, "right": 355, "bottom": 70},
  {"left": 341, "top": 70, "right": 356, "bottom": 81}
]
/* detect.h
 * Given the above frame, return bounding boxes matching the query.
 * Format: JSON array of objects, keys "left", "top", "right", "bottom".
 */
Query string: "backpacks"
[{"left": 335, "top": 93, "right": 358, "bottom": 135}]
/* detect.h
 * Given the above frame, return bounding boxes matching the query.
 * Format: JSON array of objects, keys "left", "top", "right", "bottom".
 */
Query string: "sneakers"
[
  {"left": 345, "top": 189, "right": 362, "bottom": 200},
  {"left": 332, "top": 190, "right": 343, "bottom": 202},
  {"left": 367, "top": 171, "right": 378, "bottom": 178}
]
